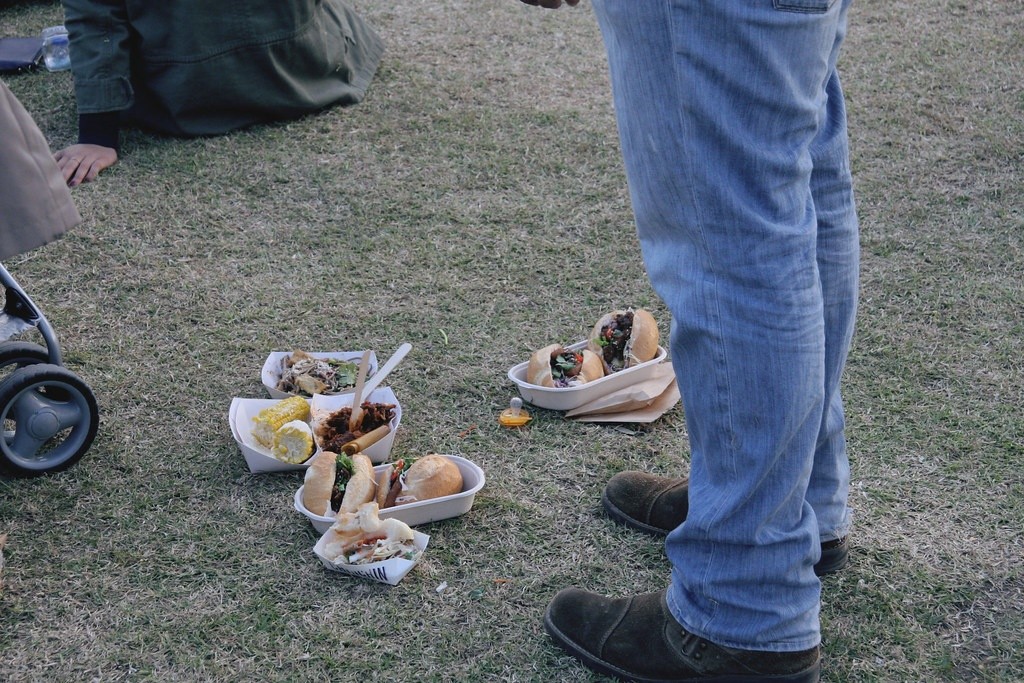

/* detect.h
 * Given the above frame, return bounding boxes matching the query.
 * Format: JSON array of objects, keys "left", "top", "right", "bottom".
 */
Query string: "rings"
[{"left": 71, "top": 157, "right": 80, "bottom": 164}]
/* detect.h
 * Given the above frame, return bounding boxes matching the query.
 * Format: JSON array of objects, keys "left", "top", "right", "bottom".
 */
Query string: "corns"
[{"left": 252, "top": 394, "right": 313, "bottom": 463}]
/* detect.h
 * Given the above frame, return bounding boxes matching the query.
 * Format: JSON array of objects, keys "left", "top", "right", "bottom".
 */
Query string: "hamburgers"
[
  {"left": 589, "top": 309, "right": 659, "bottom": 376},
  {"left": 377, "top": 456, "right": 462, "bottom": 508},
  {"left": 526, "top": 344, "right": 605, "bottom": 387},
  {"left": 303, "top": 452, "right": 375, "bottom": 519}
]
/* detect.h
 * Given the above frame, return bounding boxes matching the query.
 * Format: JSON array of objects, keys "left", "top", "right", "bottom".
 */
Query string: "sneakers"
[
  {"left": 602, "top": 470, "right": 848, "bottom": 573},
  {"left": 544, "top": 587, "right": 820, "bottom": 683}
]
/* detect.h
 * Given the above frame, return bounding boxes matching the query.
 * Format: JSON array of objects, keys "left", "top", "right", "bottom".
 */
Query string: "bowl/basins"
[
  {"left": 507, "top": 340, "right": 667, "bottom": 411},
  {"left": 293, "top": 455, "right": 486, "bottom": 533}
]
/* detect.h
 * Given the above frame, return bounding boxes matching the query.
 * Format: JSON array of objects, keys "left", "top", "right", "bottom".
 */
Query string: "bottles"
[{"left": 41, "top": 25, "right": 71, "bottom": 72}]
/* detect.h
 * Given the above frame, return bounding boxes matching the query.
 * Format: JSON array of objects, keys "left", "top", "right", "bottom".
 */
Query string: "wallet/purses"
[{"left": 1, "top": 36, "right": 43, "bottom": 73}]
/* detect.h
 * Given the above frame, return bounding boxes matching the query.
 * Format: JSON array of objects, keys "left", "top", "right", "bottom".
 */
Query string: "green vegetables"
[{"left": 324, "top": 357, "right": 360, "bottom": 386}]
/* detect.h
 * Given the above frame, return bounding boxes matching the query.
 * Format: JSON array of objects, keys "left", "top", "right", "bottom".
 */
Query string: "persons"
[
  {"left": 517, "top": 1, "right": 864, "bottom": 683},
  {"left": 49, "top": 0, "right": 386, "bottom": 186}
]
